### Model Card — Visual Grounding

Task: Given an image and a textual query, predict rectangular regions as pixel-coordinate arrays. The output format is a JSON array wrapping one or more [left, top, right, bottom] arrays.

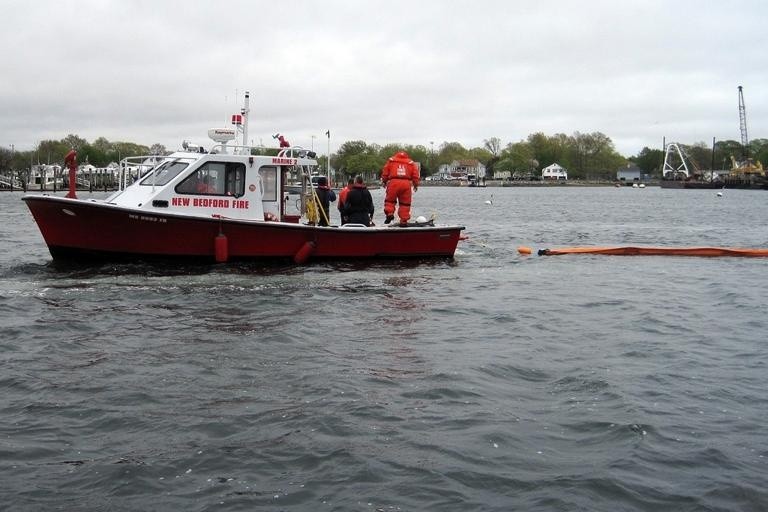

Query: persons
[[199, 175, 216, 195], [315, 150, 420, 227]]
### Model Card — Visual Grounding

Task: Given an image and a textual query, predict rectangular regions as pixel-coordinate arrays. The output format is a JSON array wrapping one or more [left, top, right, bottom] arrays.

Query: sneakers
[[384, 215, 394, 224]]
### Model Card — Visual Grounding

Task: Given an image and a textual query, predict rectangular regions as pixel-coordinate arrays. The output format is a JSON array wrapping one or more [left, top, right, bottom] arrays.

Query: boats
[[278, 172, 341, 194], [19, 88, 471, 264]]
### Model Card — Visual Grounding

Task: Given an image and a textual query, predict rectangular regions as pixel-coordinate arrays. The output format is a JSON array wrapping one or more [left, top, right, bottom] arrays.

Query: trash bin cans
[[467, 174, 476, 181]]
